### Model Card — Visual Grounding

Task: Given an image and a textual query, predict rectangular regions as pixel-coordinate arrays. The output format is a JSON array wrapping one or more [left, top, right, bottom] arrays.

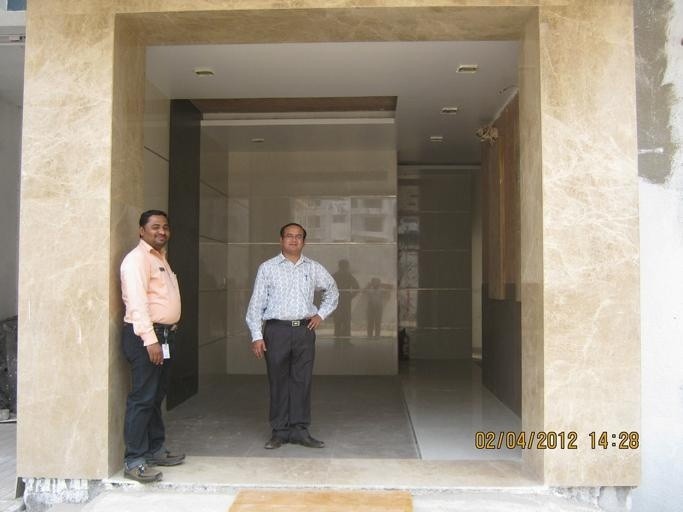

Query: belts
[[291, 321, 302, 328]]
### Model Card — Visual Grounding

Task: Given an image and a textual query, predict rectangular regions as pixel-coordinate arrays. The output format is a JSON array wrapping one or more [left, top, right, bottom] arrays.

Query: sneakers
[[289, 434, 324, 448], [146, 451, 184, 466], [264, 437, 289, 449], [125, 464, 163, 482]]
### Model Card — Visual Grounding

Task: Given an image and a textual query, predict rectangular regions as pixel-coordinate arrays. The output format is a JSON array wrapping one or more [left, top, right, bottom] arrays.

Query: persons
[[119, 209, 185, 483], [245, 222, 340, 449]]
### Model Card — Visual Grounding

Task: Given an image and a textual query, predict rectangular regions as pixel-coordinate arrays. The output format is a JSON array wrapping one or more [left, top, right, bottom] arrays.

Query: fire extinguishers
[[399, 328, 409, 360]]
